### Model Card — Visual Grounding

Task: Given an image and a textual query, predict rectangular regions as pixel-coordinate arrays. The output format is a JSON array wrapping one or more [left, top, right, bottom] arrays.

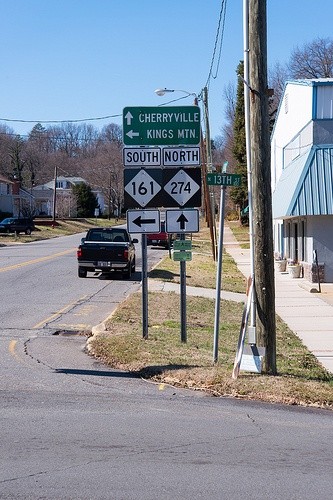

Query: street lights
[[155, 86, 216, 226]]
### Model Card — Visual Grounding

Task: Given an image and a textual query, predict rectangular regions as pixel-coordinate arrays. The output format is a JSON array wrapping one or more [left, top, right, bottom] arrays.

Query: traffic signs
[[122, 105, 203, 147]]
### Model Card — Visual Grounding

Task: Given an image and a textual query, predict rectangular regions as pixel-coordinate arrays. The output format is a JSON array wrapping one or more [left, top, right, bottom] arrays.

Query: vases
[[287, 265, 301, 278], [275, 260, 286, 272]]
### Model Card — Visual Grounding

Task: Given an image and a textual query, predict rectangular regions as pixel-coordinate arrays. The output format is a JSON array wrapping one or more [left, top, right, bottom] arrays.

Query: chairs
[[93, 233, 101, 240], [113, 236, 124, 242]]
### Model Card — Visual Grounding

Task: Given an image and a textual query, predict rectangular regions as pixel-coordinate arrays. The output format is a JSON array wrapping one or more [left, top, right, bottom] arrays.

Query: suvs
[[0, 218, 35, 236], [147, 220, 177, 248]]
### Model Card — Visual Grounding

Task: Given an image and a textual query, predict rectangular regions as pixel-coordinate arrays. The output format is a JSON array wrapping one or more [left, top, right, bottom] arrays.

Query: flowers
[[286, 259, 302, 267], [273, 250, 286, 262]]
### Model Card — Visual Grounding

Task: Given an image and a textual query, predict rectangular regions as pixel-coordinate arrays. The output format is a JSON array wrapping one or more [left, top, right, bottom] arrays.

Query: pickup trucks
[[76, 227, 139, 281]]
